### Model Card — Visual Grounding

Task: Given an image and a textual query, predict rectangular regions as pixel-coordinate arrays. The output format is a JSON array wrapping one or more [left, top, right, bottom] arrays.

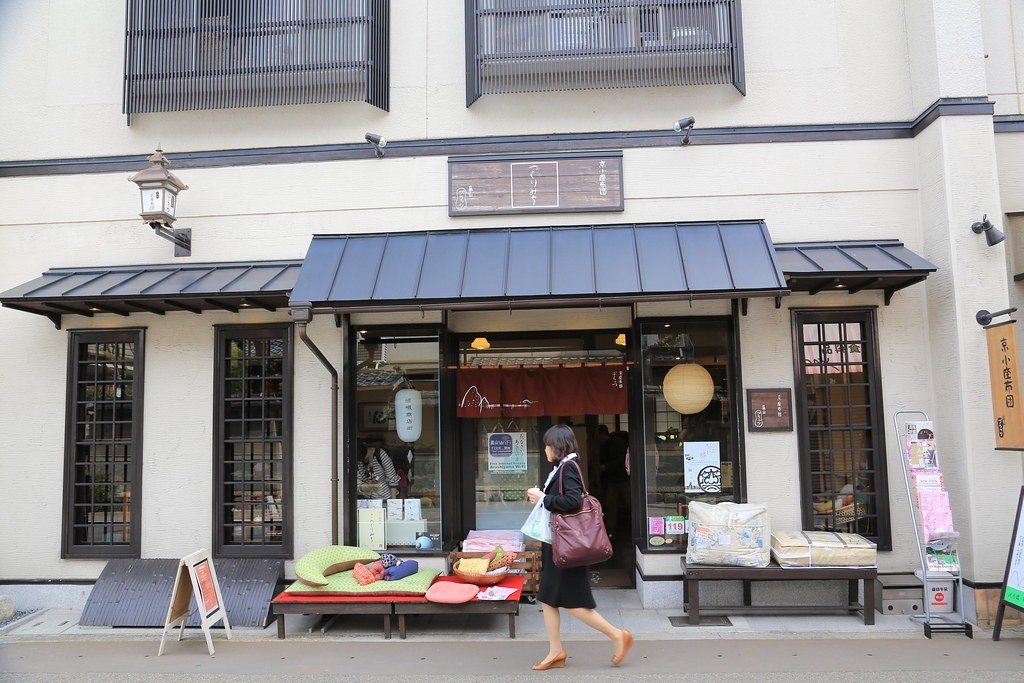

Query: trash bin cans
[[915, 569, 954, 614]]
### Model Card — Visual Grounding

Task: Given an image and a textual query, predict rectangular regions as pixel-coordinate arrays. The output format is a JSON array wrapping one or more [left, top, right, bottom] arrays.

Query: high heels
[[612, 628, 634, 665], [532, 648, 567, 671]]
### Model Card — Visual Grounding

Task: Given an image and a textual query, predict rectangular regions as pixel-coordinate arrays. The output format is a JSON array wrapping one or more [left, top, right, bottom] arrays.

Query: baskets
[[453, 556, 509, 585]]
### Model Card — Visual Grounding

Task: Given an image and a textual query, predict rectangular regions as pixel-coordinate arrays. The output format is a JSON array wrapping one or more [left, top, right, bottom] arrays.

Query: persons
[[527, 424, 633, 671], [357, 442, 401, 503], [598, 425, 627, 530]]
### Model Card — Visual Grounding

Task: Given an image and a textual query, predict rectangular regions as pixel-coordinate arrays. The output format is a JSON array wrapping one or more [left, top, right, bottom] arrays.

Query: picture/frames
[[356, 400, 397, 433]]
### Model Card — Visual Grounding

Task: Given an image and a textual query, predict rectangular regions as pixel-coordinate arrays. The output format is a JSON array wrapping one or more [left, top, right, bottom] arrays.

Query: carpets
[[588, 568, 633, 586]]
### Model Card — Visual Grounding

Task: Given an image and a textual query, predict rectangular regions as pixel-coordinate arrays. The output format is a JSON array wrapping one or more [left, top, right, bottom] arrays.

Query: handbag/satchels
[[521, 496, 554, 545], [552, 459, 614, 568]]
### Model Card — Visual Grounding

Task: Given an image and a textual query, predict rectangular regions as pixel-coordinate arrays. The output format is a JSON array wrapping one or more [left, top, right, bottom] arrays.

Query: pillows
[[294, 545, 380, 585]]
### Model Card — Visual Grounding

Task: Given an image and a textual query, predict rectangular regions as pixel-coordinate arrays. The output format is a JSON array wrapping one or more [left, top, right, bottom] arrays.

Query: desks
[[273, 600, 520, 640], [680, 556, 877, 626]]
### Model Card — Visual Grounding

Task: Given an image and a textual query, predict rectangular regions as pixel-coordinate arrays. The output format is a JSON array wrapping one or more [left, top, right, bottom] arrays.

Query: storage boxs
[[875, 576, 924, 615]]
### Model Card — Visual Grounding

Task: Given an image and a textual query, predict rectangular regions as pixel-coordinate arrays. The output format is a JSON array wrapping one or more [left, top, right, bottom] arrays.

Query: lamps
[[615, 334, 626, 346], [663, 347, 714, 415], [971, 214, 1007, 249], [365, 132, 386, 158], [470, 337, 490, 351], [127, 142, 192, 258], [671, 117, 696, 145]]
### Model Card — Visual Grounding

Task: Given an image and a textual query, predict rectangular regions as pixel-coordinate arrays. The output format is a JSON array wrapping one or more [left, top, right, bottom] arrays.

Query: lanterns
[[663, 364, 714, 414], [395, 386, 422, 446]]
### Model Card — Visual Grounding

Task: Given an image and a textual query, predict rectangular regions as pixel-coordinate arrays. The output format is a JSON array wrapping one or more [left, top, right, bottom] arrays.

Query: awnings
[[289, 219, 791, 328], [0, 258, 304, 330], [774, 238, 941, 306]]
